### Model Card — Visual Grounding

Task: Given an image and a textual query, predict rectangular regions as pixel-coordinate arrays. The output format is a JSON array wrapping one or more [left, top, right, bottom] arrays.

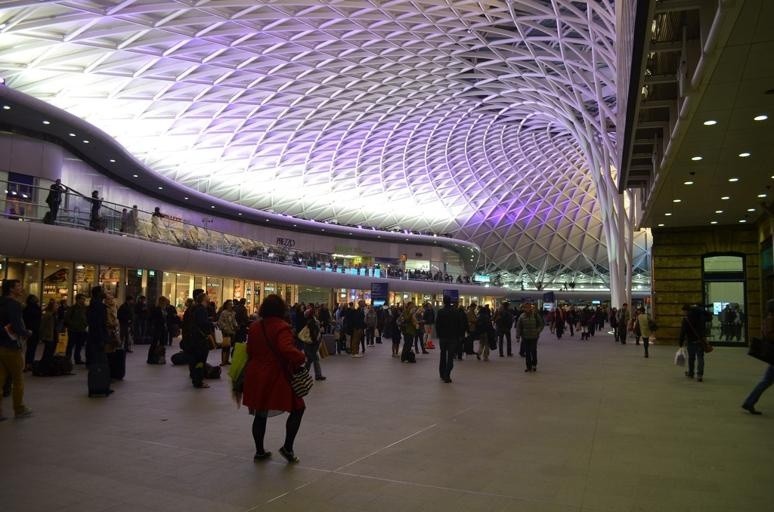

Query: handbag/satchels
[[704, 340, 713, 353], [409, 351, 415, 362], [297, 326, 313, 345], [289, 366, 314, 397]]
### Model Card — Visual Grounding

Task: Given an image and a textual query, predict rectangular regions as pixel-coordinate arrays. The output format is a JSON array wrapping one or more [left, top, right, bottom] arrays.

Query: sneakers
[[254, 451, 271, 460], [278, 446, 299, 463]]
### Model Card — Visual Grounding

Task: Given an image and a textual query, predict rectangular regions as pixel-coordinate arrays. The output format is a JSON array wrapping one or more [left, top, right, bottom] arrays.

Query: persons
[[128, 205, 137, 234], [239, 241, 471, 285], [739, 300, 773, 414], [149, 207, 168, 243], [719, 304, 744, 341], [120, 207, 129, 234], [288, 299, 436, 381], [242, 294, 305, 465], [537, 282, 541, 290], [435, 294, 545, 383], [562, 281, 568, 291], [678, 304, 713, 382], [22, 285, 250, 397], [568, 282, 572, 289], [547, 301, 657, 358], [572, 281, 575, 289], [90, 190, 103, 231], [44, 179, 67, 225], [519, 278, 524, 290], [2, 278, 34, 421]]
